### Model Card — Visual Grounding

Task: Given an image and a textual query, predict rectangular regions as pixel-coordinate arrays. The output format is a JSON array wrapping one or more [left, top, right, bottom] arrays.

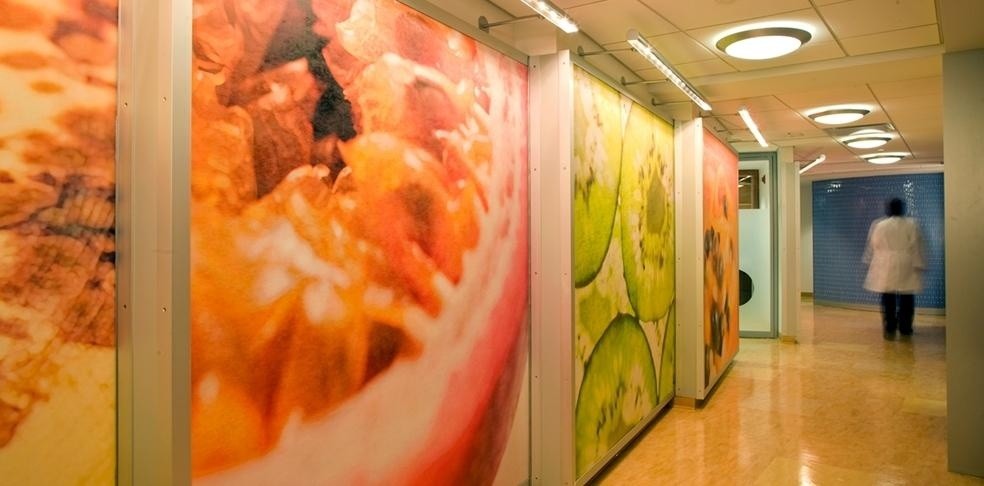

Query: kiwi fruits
[[572, 65, 676, 479]]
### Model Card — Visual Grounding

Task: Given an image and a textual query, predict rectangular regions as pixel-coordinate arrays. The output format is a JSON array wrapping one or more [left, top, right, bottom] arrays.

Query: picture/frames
[[737, 169, 758, 210]]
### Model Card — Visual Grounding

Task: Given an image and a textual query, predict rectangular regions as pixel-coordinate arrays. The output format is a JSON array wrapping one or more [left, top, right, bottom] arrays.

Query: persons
[[863, 197, 928, 341]]
